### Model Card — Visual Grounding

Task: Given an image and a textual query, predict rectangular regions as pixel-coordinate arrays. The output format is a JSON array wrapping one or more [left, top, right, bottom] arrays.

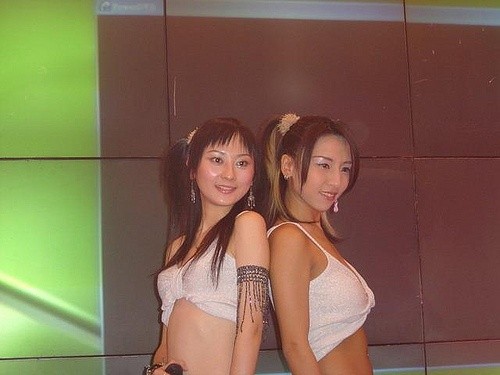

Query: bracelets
[[146, 362, 165, 375]]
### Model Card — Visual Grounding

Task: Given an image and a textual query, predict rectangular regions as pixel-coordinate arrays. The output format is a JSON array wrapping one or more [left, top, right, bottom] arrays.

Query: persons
[[143, 116, 270, 375], [257, 110, 377, 375]]
